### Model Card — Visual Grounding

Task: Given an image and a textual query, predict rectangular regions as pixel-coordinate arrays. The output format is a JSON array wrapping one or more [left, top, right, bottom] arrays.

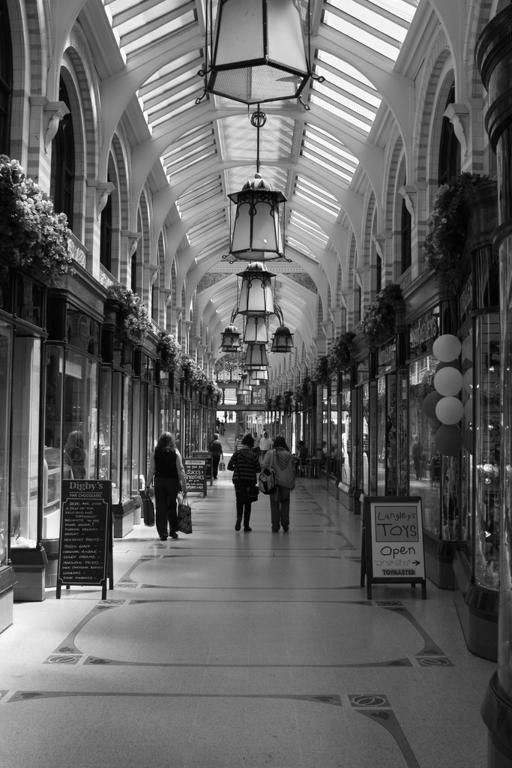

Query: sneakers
[[235, 518, 241, 531], [169, 529, 177, 537], [273, 525, 289, 533], [244, 527, 251, 532], [160, 532, 169, 540]]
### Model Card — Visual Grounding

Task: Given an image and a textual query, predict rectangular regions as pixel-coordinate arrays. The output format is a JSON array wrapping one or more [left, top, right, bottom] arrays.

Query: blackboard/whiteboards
[[366, 497, 427, 582], [183, 451, 213, 492], [57, 479, 111, 585]]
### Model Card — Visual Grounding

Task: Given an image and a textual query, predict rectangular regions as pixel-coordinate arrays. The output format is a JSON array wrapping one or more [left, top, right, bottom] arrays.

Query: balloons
[[432, 333, 462, 365], [421, 362, 463, 458]]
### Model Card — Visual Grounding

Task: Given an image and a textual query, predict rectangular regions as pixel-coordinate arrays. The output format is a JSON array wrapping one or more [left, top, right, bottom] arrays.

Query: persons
[[220, 419, 225, 436], [294, 440, 308, 476], [233, 428, 273, 460], [215, 417, 220, 433], [227, 433, 261, 533], [207, 433, 224, 480], [142, 430, 188, 542], [63, 430, 86, 481], [411, 436, 427, 481], [261, 435, 297, 534], [321, 440, 330, 470]]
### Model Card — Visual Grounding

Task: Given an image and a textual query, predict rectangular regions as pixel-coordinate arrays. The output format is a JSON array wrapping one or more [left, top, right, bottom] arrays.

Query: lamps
[[226, 105, 288, 263], [191, 0, 329, 105], [219, 265, 295, 392]]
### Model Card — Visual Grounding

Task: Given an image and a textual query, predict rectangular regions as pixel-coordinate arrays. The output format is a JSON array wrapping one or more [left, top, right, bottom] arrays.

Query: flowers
[[263, 295, 399, 403], [419, 169, 499, 279], [103, 281, 224, 405], [2, 151, 77, 285]]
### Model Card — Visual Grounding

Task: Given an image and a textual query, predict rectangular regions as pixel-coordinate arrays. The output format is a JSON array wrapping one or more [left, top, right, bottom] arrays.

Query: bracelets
[[145, 486, 150, 488]]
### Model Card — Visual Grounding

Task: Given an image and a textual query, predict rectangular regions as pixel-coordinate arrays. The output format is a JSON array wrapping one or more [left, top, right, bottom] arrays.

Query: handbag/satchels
[[175, 504, 192, 535], [236, 479, 257, 498], [143, 500, 155, 526], [259, 468, 277, 495]]
